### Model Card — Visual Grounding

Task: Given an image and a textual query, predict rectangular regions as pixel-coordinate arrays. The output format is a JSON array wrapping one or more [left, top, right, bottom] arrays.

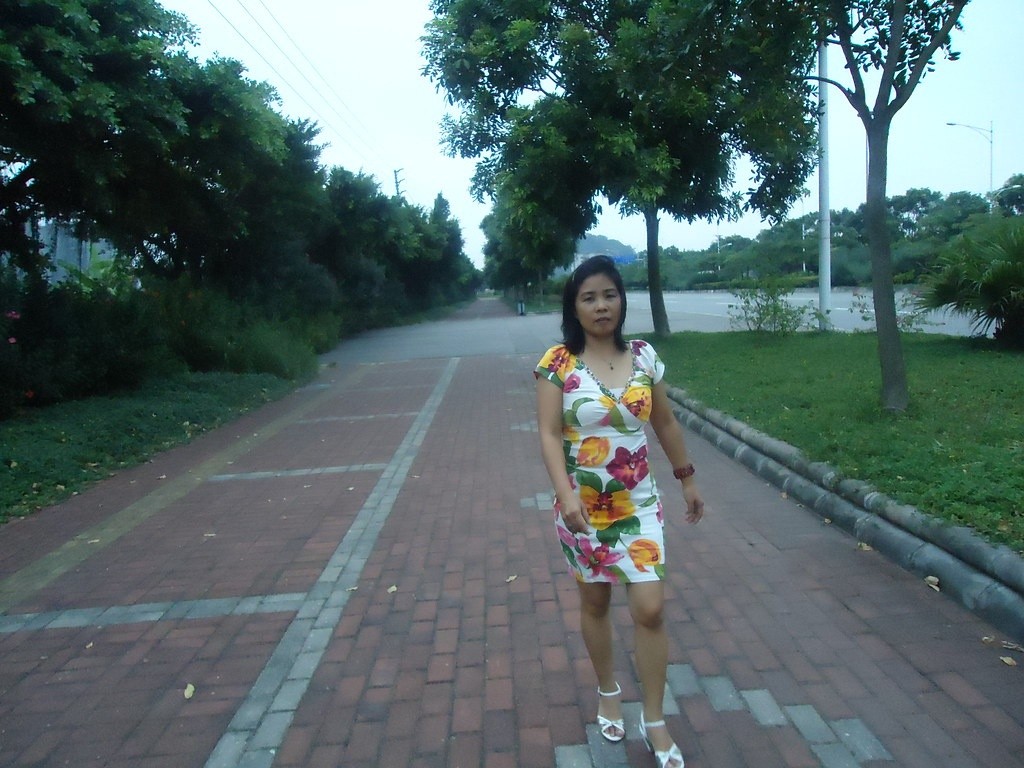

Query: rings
[[567, 524, 572, 527]]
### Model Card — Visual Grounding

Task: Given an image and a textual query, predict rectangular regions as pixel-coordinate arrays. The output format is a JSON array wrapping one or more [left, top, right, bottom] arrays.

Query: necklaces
[[588, 346, 617, 370]]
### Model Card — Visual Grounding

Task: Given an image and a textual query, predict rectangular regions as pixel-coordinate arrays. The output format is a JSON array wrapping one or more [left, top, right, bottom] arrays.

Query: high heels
[[597, 682, 626, 742], [638, 711, 684, 768]]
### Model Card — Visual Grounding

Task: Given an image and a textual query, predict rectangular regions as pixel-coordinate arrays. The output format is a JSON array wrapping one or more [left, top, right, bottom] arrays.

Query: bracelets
[[673, 462, 696, 479]]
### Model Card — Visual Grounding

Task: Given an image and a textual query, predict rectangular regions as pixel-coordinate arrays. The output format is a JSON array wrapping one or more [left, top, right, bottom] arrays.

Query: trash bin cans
[[518, 300, 525, 316]]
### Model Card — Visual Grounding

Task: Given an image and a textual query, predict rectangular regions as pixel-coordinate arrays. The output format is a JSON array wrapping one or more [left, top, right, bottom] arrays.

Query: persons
[[533, 255, 706, 768]]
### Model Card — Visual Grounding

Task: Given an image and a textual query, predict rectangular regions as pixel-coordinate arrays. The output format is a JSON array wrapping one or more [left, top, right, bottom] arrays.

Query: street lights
[[713, 235, 733, 270], [946, 119, 994, 221]]
